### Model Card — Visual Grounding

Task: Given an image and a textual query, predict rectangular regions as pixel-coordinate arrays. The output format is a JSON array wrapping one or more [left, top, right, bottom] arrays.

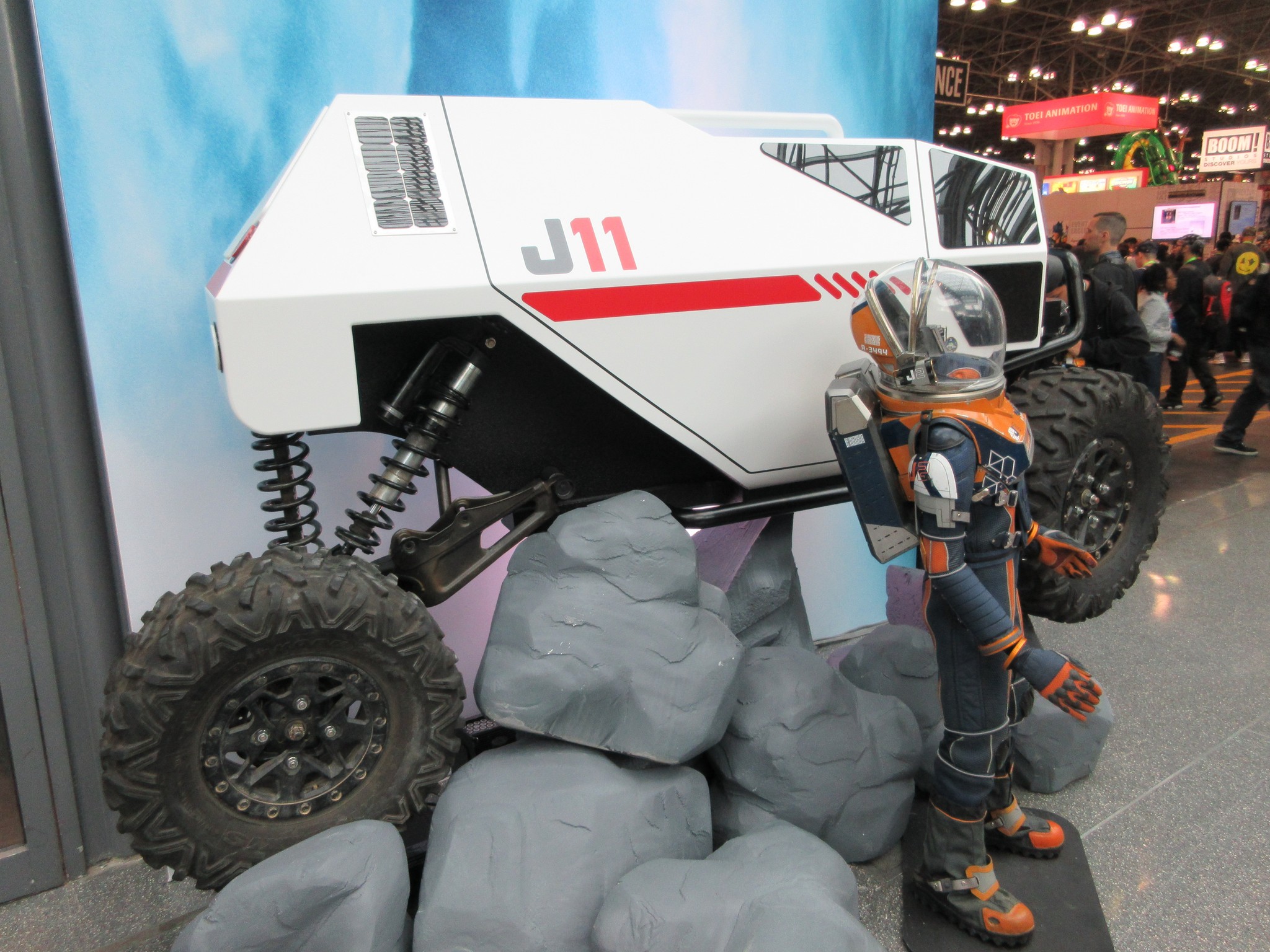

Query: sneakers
[[1212, 431, 1258, 456]]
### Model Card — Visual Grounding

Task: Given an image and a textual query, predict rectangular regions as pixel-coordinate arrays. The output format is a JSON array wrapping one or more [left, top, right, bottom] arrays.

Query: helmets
[[849, 257, 1008, 394]]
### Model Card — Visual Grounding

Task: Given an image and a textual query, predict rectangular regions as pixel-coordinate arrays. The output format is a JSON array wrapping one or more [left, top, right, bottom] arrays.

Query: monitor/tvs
[[1228, 201, 1257, 236], [1149, 202, 1216, 239]]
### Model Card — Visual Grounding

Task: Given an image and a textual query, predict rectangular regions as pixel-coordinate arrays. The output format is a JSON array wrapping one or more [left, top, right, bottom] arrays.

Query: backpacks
[[1180, 261, 1233, 333]]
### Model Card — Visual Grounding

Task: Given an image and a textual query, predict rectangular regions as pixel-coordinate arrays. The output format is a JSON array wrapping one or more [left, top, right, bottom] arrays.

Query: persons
[[824, 257, 1102, 947], [1044, 211, 1270, 456]]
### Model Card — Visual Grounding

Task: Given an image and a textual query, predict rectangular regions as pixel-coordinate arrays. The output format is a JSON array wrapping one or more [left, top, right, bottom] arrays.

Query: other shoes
[[1157, 395, 1184, 409], [1198, 389, 1224, 407], [1208, 352, 1226, 364], [1237, 352, 1250, 363]]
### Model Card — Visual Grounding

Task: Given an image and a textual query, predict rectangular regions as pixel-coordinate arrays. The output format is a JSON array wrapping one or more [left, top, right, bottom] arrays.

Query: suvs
[[92, 86, 1173, 897]]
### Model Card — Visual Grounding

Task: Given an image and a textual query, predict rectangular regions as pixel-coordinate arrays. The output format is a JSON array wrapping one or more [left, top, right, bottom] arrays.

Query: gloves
[[1025, 521, 1098, 579], [1003, 637, 1103, 722]]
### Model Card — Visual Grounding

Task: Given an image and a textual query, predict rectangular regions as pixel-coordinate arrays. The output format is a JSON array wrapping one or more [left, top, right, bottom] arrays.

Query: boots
[[984, 762, 1064, 860], [912, 796, 1035, 947]]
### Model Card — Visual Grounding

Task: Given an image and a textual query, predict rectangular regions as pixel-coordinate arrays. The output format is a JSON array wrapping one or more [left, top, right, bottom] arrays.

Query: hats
[[1242, 227, 1257, 236], [1176, 234, 1206, 247], [1134, 239, 1158, 254]]
